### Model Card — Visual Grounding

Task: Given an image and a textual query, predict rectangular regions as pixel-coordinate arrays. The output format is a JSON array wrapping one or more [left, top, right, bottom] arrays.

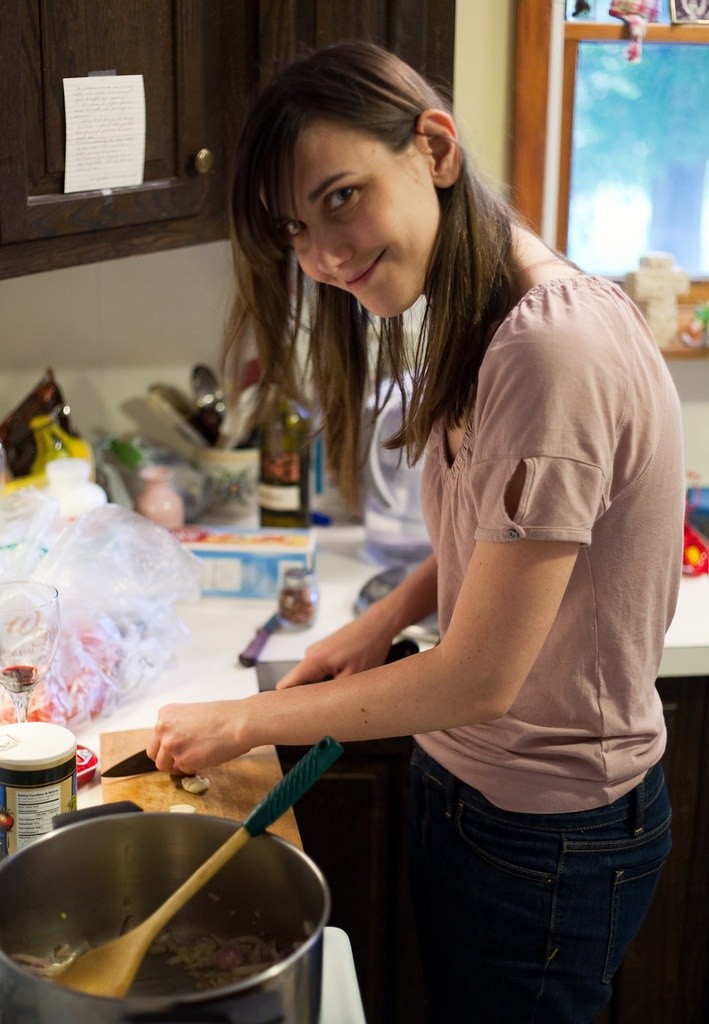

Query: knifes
[[239, 614, 279, 667], [99, 747, 158, 777]]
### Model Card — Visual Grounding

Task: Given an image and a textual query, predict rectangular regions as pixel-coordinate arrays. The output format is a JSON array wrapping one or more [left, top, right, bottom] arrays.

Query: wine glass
[[0, 582, 62, 722]]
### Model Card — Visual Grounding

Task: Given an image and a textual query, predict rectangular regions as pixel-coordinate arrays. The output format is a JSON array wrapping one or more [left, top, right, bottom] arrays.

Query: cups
[[195, 450, 262, 517]]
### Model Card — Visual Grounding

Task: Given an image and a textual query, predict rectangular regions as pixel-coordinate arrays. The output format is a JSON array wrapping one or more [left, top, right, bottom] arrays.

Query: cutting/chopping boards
[[98, 727, 303, 857]]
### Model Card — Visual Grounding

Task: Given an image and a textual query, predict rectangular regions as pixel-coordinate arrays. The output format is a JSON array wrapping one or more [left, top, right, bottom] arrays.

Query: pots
[[0, 800, 332, 1023]]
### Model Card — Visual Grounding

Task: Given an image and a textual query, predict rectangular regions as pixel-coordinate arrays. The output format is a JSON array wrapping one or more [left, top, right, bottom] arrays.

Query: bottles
[[258, 404, 308, 531], [280, 567, 317, 632]]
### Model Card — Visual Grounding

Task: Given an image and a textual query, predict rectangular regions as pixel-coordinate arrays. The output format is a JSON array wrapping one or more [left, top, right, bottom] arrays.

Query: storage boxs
[[169, 525, 319, 599]]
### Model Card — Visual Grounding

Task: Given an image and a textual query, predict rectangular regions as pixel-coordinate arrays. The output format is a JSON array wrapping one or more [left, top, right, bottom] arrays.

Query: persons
[[143, 39, 687, 1024]]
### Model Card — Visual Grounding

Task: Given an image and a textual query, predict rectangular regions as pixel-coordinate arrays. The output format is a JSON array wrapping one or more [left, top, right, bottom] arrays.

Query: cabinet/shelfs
[[276, 672, 709, 1024], [0, 1, 456, 281]]
